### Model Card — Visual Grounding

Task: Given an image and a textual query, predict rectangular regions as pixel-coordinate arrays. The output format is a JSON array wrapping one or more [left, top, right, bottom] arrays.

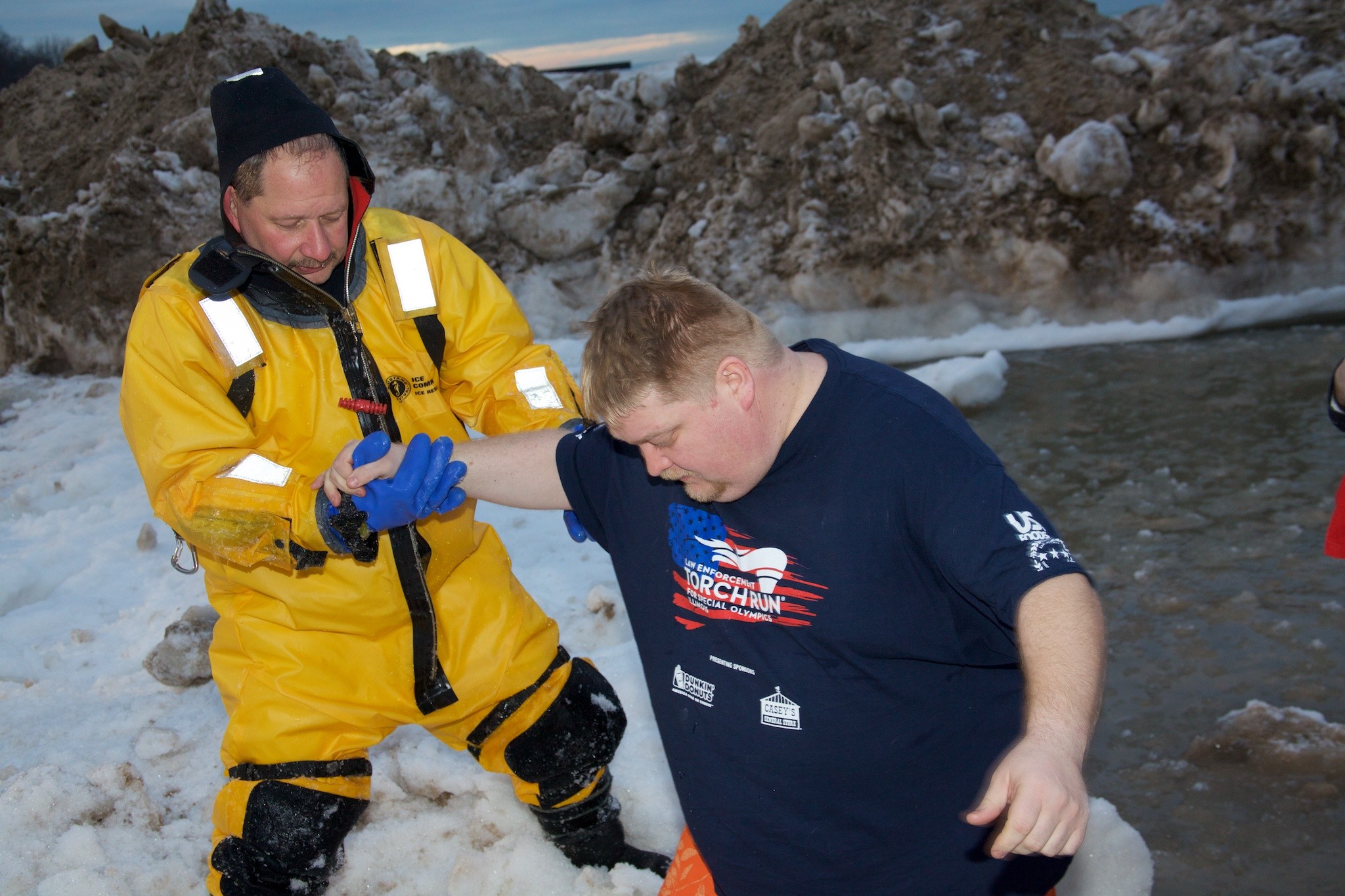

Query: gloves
[[317, 431, 468, 554]]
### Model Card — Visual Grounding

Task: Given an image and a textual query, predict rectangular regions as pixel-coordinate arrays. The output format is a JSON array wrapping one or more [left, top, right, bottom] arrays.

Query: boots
[[531, 772, 672, 880]]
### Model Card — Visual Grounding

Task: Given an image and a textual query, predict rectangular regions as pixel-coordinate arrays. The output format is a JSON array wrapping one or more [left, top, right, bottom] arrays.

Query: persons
[[116, 57, 607, 895], [305, 263, 1113, 895]]
